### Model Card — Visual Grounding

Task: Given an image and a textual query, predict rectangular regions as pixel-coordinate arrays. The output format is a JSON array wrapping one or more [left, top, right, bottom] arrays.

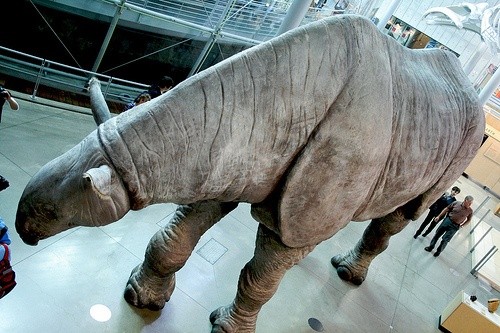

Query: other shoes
[[413, 233, 418, 239], [422, 233, 427, 237], [433, 252, 440, 257], [425, 247, 433, 252]]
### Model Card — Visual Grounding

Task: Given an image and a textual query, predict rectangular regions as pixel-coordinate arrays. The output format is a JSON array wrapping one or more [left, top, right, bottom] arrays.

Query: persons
[[424, 196, 474, 257], [123, 76, 174, 109], [414, 186, 461, 240], [0, 71, 18, 298]]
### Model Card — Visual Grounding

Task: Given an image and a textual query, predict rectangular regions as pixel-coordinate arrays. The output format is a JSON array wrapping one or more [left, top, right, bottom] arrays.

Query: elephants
[[15, 15, 486, 333]]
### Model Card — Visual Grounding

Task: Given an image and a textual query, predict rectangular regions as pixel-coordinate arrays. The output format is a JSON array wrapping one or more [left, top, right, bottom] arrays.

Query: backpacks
[[0, 243, 17, 299]]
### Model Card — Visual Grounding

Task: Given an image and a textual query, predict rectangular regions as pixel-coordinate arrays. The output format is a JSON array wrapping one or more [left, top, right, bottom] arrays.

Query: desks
[[438, 290, 500, 333]]
